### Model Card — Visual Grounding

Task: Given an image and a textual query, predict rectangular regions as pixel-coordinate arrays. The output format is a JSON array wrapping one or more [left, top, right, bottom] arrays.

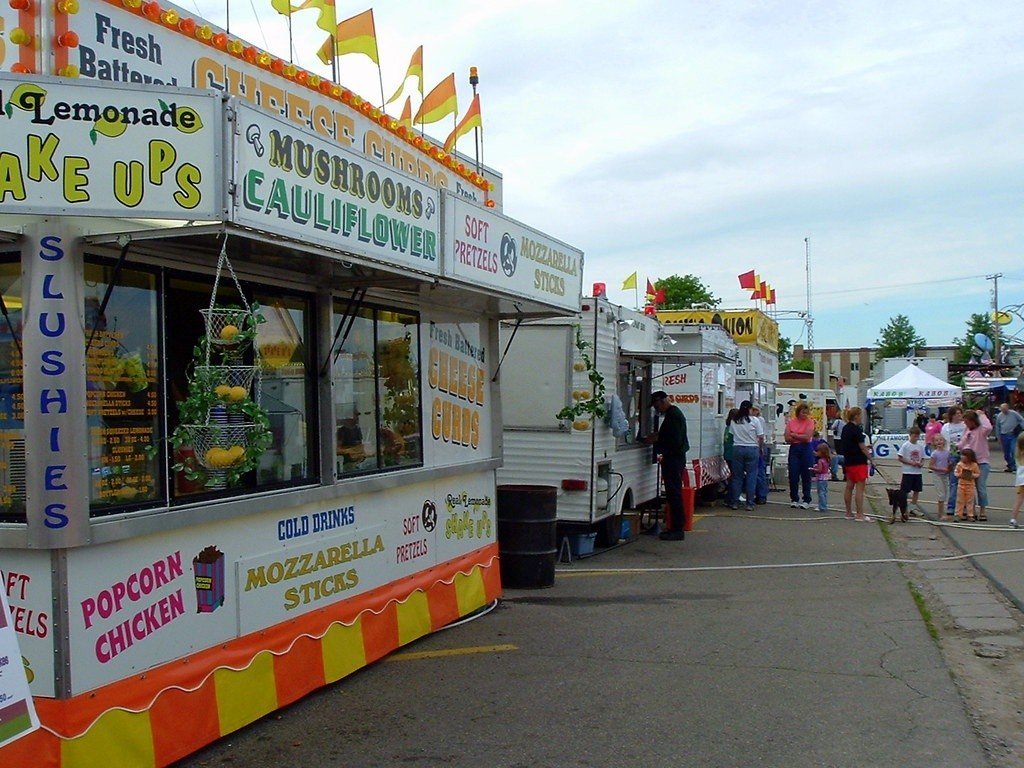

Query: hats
[[647, 390, 668, 410], [753, 401, 761, 411], [740, 401, 753, 409]]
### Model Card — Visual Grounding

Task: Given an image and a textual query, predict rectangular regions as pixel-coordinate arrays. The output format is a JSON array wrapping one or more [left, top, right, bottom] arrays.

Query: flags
[[414, 73, 458, 126], [965, 356, 983, 378], [443, 93, 483, 154], [271, 0, 301, 16], [399, 96, 411, 126], [297, 0, 336, 38], [317, 9, 379, 66], [378, 46, 423, 109]]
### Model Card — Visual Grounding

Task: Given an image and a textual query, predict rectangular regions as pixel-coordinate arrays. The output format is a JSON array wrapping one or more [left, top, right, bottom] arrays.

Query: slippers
[[844, 514, 857, 520], [854, 516, 875, 522]]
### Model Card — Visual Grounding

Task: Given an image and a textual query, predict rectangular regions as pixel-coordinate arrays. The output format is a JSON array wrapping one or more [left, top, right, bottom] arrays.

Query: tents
[[867, 363, 962, 436]]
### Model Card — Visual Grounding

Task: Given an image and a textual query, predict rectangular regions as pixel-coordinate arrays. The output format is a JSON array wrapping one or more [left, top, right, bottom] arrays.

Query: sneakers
[[801, 502, 810, 509], [790, 501, 799, 507]]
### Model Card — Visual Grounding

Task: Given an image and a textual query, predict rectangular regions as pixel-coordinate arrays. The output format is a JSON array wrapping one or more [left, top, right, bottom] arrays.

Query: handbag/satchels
[[834, 430, 838, 435]]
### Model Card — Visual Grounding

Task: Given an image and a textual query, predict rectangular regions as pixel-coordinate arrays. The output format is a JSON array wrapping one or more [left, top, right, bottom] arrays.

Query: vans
[[776, 388, 842, 448]]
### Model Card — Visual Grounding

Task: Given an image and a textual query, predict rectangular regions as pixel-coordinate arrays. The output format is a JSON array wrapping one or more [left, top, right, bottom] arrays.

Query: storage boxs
[[574, 531, 596, 555], [621, 511, 641, 538], [619, 521, 629, 540]]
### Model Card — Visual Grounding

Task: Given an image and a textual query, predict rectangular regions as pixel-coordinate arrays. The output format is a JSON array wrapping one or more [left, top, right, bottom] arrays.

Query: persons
[[397, 418, 419, 460], [723, 400, 768, 511], [784, 404, 874, 522], [896, 403, 1024, 527], [335, 408, 366, 472], [643, 391, 690, 541]]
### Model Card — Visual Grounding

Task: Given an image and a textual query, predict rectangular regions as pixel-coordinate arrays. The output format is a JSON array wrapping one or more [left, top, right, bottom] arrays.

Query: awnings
[[1006, 384, 1018, 390], [962, 384, 1004, 393]]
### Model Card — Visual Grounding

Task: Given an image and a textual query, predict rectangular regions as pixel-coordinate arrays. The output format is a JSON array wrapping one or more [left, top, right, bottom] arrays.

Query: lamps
[[661, 334, 678, 348], [608, 313, 634, 333]]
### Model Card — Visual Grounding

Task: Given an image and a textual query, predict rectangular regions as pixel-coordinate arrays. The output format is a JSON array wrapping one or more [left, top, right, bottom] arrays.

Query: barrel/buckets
[[666, 488, 695, 531], [496, 484, 559, 588]]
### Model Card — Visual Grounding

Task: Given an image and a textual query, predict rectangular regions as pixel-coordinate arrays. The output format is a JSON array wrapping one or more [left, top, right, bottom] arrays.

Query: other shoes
[[1004, 468, 1013, 473], [831, 476, 839, 481], [746, 504, 757, 510], [939, 511, 988, 522], [1009, 518, 1019, 527], [659, 529, 686, 541], [731, 502, 740, 509], [813, 507, 820, 511], [755, 498, 767, 505], [909, 509, 925, 518]]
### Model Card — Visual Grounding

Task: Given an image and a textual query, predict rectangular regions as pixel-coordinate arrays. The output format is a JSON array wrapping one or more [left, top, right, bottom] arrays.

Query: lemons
[[221, 325, 240, 340], [574, 363, 586, 371], [572, 421, 589, 431], [206, 445, 245, 467], [215, 385, 248, 400], [573, 390, 591, 400]]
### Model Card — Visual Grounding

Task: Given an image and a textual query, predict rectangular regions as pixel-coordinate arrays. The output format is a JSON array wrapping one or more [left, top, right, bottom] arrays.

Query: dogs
[[884, 488, 908, 524]]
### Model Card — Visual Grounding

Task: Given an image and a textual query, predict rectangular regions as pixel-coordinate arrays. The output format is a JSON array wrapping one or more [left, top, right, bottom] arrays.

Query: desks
[[871, 432, 931, 458]]
